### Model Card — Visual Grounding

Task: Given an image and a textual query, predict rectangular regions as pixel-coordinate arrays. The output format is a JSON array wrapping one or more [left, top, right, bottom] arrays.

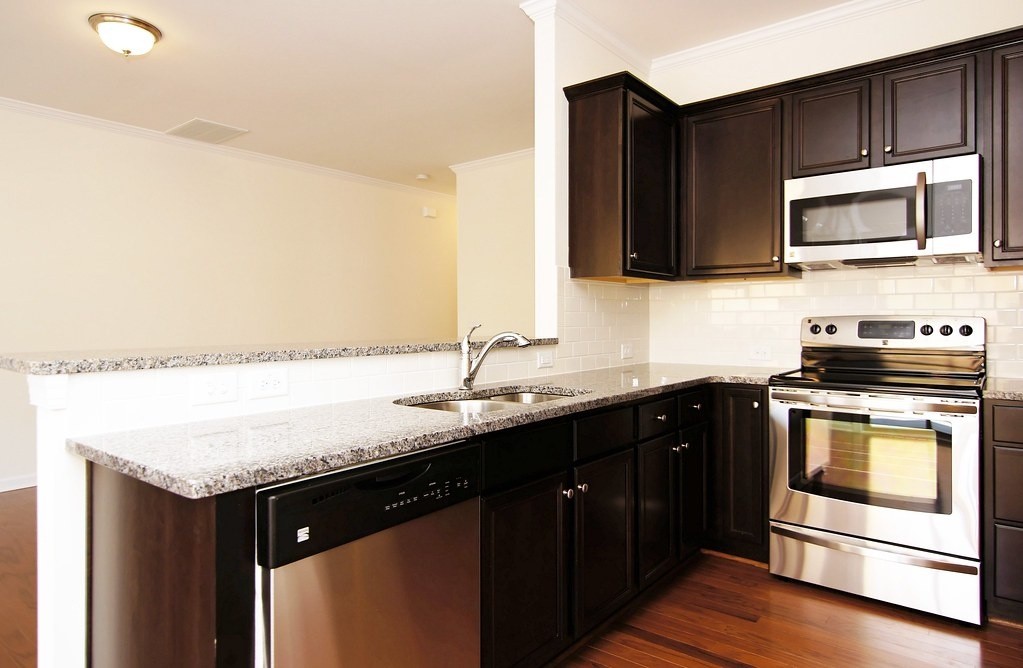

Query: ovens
[[768, 386, 980, 625]]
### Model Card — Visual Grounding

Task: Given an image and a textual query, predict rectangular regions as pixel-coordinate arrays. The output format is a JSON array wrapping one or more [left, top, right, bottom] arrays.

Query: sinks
[[402, 400, 519, 421], [490, 392, 578, 405]]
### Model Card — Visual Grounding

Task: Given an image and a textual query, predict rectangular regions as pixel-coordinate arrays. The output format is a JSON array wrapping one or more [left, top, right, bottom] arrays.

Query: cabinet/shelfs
[[491, 401, 638, 668], [680, 76, 802, 282], [983, 400, 1023, 618], [704, 383, 769, 564], [787, 32, 992, 179], [563, 70, 679, 283], [638, 384, 704, 598], [984, 25, 1023, 267]]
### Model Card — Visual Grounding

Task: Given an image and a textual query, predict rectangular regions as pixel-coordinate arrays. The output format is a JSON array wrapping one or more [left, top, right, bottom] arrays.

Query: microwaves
[[784, 154, 984, 264]]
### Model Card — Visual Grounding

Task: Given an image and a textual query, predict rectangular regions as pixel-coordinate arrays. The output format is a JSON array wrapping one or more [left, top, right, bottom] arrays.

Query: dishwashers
[[249, 437, 481, 668]]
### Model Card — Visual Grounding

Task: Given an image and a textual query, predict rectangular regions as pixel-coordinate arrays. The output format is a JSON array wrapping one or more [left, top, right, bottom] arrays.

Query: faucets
[[458, 324, 531, 390]]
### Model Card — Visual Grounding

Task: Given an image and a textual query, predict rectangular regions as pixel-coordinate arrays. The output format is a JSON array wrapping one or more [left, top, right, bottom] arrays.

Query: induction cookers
[[769, 315, 987, 399]]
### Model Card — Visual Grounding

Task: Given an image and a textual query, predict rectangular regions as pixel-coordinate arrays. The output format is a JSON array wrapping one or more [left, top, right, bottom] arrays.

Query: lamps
[[88, 13, 162, 58]]
